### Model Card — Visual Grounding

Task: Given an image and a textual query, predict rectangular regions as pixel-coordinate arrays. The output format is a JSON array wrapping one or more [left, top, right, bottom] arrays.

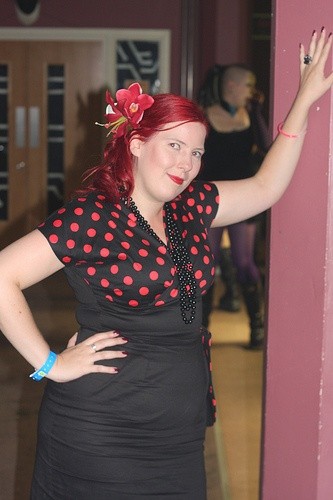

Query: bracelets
[[278, 124, 298, 138], [29, 351, 57, 382]]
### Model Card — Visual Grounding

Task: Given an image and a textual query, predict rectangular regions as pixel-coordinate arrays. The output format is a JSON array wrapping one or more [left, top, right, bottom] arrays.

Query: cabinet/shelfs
[[0, 28, 197, 300]]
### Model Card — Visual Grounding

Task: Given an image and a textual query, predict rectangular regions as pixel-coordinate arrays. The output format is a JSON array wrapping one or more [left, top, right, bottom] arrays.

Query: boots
[[239, 278, 265, 350], [214, 246, 240, 314], [202, 284, 213, 328]]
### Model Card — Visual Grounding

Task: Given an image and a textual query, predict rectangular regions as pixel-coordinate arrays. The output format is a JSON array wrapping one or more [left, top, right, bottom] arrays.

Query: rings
[[304, 56, 312, 65], [92, 344, 96, 350]]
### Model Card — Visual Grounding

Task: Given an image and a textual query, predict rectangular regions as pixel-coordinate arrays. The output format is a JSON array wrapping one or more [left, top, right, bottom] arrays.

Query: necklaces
[[118, 185, 197, 324]]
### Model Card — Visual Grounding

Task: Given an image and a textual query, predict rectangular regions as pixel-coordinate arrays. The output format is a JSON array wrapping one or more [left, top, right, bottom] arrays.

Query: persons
[[198, 63, 265, 349], [0, 25, 333, 500]]
